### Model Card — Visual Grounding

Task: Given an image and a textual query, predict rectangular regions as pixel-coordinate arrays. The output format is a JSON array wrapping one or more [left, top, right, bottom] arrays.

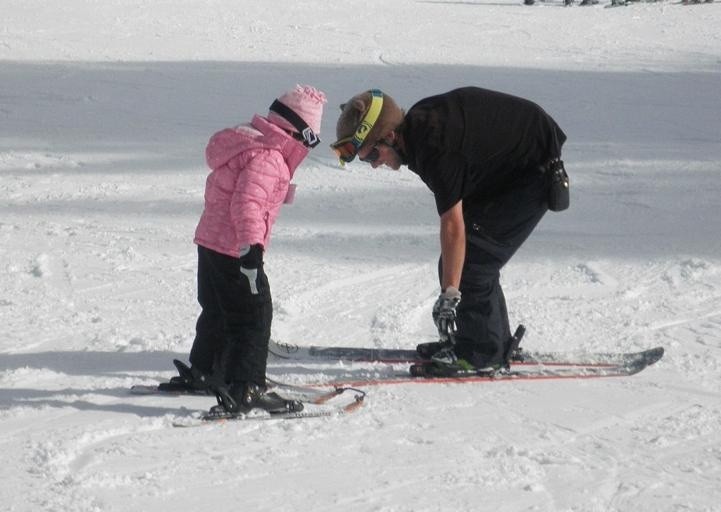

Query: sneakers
[[188, 365, 211, 390], [228, 382, 304, 415], [417, 342, 509, 375]]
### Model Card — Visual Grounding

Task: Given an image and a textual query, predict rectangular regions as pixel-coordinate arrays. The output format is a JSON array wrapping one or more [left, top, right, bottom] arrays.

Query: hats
[[336, 92, 403, 142], [267, 87, 326, 138]]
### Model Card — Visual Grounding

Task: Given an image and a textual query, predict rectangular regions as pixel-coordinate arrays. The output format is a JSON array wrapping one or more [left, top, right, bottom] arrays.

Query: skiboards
[[131, 385, 365, 428], [266, 341, 664, 386]]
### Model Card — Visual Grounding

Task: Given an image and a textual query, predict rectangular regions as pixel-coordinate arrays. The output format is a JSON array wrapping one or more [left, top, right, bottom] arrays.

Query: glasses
[[360, 140, 382, 164], [268, 98, 321, 151], [333, 90, 384, 166]]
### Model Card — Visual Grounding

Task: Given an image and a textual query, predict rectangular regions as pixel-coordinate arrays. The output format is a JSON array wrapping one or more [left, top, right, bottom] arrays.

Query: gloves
[[241, 244, 262, 269], [431, 287, 460, 344]]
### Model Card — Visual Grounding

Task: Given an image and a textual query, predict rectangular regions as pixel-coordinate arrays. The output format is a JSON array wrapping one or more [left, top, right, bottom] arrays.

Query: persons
[[332, 86, 568, 375], [171, 82, 327, 417]]
